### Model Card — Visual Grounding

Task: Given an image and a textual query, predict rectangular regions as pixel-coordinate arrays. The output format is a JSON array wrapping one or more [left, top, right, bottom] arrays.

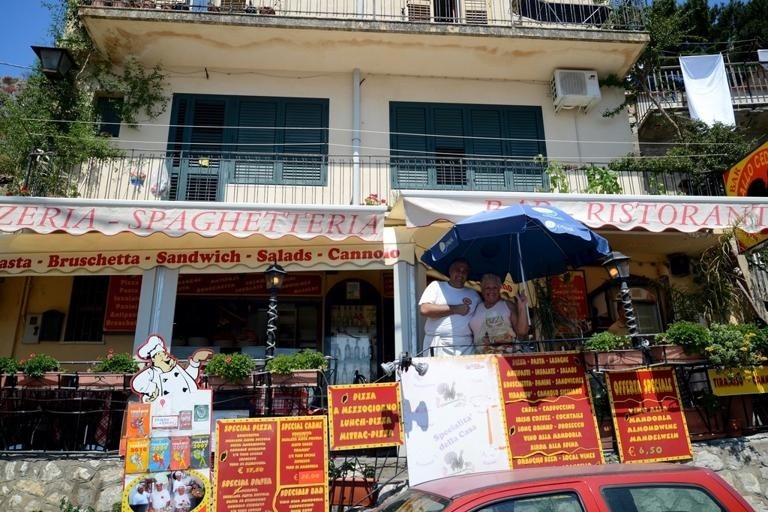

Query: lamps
[[30, 45, 81, 104]]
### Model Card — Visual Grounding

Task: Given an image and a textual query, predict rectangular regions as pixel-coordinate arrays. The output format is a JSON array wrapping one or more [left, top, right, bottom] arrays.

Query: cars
[[344, 462, 756, 512]]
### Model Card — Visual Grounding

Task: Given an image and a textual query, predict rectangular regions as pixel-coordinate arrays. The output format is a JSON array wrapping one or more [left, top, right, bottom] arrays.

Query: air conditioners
[[546, 68, 601, 115]]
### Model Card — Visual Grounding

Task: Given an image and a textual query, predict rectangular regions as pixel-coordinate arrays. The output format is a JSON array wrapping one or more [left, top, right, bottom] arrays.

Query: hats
[[156, 481, 163, 485], [175, 471, 182, 476], [138, 336, 167, 360]]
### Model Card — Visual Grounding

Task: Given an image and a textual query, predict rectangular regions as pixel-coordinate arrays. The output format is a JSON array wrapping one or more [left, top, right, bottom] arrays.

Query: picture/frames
[[101, 275, 142, 335]]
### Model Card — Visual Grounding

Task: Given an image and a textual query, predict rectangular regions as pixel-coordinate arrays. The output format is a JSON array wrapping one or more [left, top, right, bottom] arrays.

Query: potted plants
[[650, 320, 768, 378], [328, 460, 379, 507], [265, 348, 329, 389], [583, 331, 643, 374], [0, 354, 18, 388]]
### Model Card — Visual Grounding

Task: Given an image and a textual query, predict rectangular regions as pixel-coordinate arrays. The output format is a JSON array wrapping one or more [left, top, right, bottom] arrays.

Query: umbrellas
[[421, 204, 609, 342]]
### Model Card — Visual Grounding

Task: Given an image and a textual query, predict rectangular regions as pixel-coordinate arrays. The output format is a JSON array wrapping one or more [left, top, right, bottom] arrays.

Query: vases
[[207, 3, 276, 14], [90, 0, 190, 10], [77, 371, 125, 391], [16, 369, 60, 388], [208, 374, 253, 390]]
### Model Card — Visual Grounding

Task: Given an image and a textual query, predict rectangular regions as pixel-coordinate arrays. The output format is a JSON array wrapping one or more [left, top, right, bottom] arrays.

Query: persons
[[128, 471, 191, 512], [131, 334, 213, 404], [467, 272, 529, 355], [416, 258, 481, 357], [608, 302, 629, 336]]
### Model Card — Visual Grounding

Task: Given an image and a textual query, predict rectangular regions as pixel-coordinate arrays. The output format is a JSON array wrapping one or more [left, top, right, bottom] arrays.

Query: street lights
[[263, 258, 287, 416], [601, 246, 644, 349]]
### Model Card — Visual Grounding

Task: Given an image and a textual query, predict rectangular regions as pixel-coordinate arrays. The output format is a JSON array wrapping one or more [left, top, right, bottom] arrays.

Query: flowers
[[202, 351, 255, 381], [76, 352, 139, 374], [13, 353, 66, 374]]
